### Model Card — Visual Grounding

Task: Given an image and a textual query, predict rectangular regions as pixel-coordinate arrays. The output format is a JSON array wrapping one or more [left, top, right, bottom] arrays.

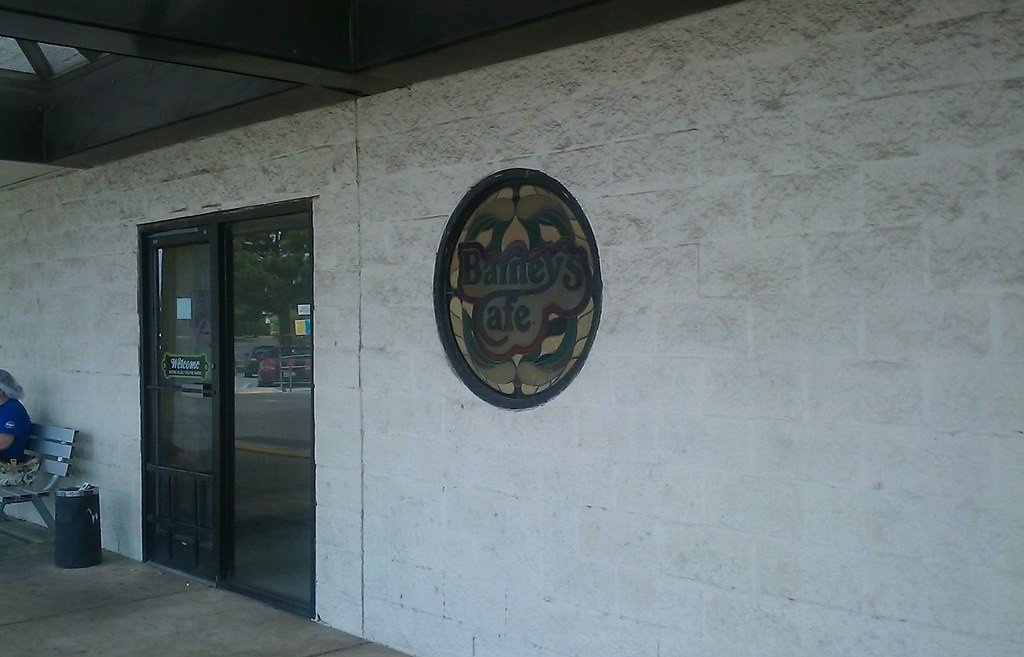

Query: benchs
[[0, 420, 81, 536]]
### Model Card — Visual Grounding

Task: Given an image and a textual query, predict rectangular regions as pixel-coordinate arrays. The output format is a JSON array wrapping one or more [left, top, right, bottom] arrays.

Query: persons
[[0, 369, 32, 487]]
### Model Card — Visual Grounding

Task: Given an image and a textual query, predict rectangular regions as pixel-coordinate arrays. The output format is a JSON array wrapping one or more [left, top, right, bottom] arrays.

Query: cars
[[240, 344, 314, 387]]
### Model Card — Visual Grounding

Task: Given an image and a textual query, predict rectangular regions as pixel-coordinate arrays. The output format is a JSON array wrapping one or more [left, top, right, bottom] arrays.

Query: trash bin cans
[[54, 483, 102, 570]]
